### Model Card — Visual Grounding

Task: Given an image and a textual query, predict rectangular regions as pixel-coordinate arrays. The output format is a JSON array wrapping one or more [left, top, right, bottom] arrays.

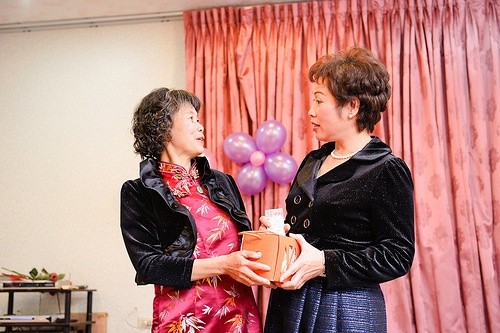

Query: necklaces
[[331, 139, 371, 159], [197, 187, 202, 192]]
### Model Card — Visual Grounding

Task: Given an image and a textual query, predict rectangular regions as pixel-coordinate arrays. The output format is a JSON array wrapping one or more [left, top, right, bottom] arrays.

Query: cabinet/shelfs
[[0, 285, 97, 333]]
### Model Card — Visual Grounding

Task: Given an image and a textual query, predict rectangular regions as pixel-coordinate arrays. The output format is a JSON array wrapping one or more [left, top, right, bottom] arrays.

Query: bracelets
[[320, 251, 326, 277]]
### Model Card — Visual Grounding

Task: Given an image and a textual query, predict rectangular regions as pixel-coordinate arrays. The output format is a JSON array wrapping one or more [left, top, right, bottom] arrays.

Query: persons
[[120, 88, 276, 333], [258, 47, 415, 333]]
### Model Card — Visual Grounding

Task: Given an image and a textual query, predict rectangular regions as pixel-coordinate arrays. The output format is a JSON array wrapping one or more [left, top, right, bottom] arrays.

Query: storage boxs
[[240, 231, 299, 284]]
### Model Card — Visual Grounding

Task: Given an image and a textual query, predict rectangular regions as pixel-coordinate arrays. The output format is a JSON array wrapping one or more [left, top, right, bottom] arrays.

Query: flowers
[[1, 266, 65, 282]]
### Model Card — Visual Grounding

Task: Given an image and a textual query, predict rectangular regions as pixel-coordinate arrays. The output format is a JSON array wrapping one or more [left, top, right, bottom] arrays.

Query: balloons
[[236, 163, 267, 195], [200, 148, 215, 168], [255, 119, 286, 154], [250, 151, 265, 165], [264, 153, 298, 184], [225, 131, 256, 163]]
